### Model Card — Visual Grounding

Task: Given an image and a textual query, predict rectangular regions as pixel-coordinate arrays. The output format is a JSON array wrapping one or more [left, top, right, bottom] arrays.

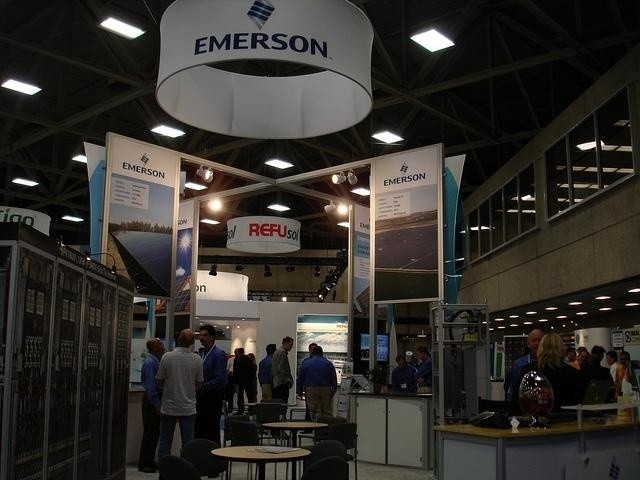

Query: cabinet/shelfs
[[1, 220, 137, 480], [428, 299, 491, 477]]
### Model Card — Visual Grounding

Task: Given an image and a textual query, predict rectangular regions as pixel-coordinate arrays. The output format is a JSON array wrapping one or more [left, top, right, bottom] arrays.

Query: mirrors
[[519, 369, 555, 432]]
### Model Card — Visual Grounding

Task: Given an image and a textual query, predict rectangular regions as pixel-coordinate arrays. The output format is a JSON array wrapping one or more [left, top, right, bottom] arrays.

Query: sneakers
[[139, 462, 158, 473]]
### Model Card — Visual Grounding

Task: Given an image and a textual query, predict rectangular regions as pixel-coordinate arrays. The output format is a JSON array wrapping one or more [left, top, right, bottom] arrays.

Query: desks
[[433, 413, 640, 480]]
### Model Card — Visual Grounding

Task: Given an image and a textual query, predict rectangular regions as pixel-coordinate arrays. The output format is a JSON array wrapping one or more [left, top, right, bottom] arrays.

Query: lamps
[[208, 261, 342, 302], [329, 166, 358, 187], [323, 196, 337, 213], [194, 162, 216, 183]]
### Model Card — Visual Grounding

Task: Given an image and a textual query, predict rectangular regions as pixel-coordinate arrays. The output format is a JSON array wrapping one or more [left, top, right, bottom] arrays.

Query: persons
[[137, 324, 338, 474], [503, 328, 637, 415], [389, 346, 432, 397]]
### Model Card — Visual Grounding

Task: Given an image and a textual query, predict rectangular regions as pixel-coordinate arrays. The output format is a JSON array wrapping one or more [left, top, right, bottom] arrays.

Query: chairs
[[156, 395, 362, 480]]
